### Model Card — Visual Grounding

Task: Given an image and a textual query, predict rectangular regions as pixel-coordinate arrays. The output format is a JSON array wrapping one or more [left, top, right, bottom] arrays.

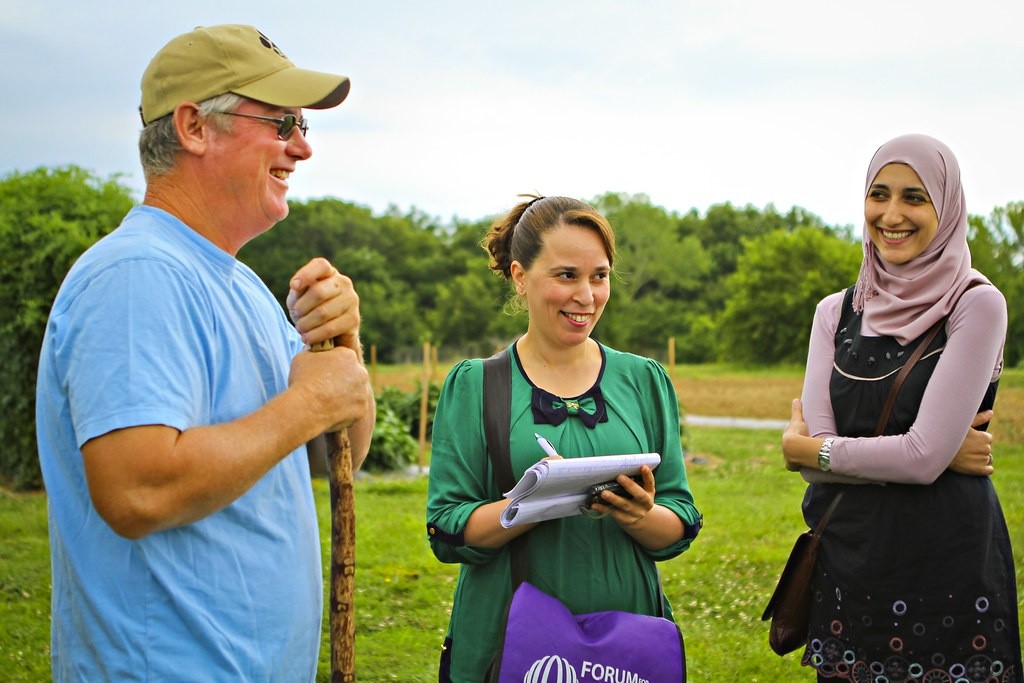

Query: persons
[[780, 134, 1024, 683], [427, 195, 703, 683], [37, 24, 377, 683]]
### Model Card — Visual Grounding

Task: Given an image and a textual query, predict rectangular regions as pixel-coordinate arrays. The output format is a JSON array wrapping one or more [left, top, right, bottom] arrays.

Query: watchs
[[818, 437, 834, 472]]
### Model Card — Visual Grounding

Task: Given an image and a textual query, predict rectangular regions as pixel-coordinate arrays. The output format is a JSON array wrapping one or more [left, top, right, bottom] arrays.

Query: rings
[[988, 454, 993, 465]]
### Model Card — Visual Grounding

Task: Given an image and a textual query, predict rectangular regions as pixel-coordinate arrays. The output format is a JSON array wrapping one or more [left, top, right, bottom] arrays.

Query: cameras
[[584, 476, 641, 510]]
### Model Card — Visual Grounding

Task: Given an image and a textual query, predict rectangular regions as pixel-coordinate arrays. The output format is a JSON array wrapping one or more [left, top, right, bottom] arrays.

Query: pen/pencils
[[533, 431, 558, 458]]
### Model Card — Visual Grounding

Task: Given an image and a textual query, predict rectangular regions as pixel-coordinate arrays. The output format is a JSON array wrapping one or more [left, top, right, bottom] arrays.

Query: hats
[[139, 25, 350, 128]]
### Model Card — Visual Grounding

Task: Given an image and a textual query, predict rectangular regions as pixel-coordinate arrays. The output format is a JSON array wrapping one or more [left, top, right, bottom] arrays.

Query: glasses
[[206, 110, 308, 141]]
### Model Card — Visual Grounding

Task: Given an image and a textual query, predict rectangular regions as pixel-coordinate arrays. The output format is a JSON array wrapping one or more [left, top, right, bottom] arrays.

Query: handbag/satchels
[[762, 530, 821, 657], [483, 582, 686, 683]]
